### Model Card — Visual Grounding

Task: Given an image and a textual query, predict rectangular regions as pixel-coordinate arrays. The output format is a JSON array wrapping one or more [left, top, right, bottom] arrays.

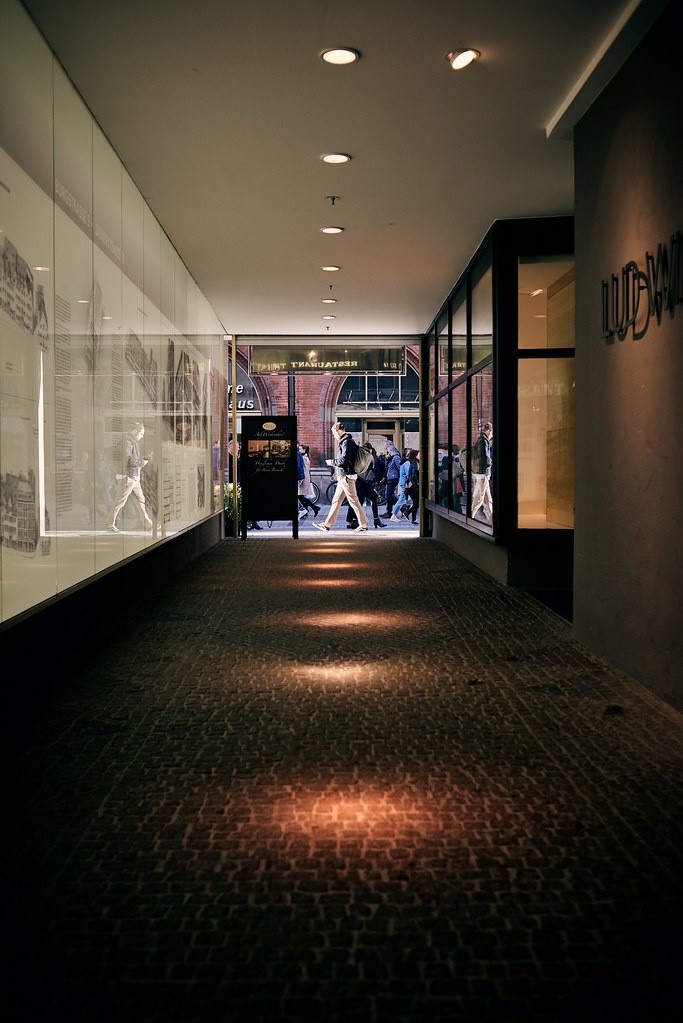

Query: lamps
[[449, 49, 475, 70]]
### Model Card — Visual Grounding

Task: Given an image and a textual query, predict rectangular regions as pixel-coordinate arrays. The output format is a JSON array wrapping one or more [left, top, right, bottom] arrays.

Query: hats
[[362, 443, 372, 449]]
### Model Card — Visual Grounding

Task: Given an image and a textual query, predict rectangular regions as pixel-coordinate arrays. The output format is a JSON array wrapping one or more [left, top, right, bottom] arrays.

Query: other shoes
[[314, 506, 322, 517], [347, 522, 357, 528], [410, 520, 419, 525], [402, 512, 410, 522], [379, 512, 393, 520]]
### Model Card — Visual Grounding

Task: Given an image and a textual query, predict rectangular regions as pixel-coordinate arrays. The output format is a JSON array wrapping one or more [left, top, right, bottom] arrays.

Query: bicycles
[[325, 480, 389, 507], [299, 480, 320, 506]]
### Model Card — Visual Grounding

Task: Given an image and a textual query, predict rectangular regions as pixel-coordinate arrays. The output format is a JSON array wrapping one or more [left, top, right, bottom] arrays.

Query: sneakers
[[356, 522, 368, 531], [391, 514, 401, 523], [311, 522, 329, 532], [299, 509, 308, 519]]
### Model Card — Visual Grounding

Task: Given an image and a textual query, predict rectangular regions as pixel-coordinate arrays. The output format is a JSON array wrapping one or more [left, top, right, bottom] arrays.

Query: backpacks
[[353, 444, 374, 475]]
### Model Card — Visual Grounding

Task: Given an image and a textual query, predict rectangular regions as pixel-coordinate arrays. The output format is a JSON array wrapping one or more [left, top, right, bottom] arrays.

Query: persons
[[346, 439, 420, 530], [211, 438, 321, 531], [439, 422, 493, 520], [105, 422, 162, 532], [312, 422, 370, 532]]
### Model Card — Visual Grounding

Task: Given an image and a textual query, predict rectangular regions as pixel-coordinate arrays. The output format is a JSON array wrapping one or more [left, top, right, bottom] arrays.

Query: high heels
[[374, 518, 387, 528]]
